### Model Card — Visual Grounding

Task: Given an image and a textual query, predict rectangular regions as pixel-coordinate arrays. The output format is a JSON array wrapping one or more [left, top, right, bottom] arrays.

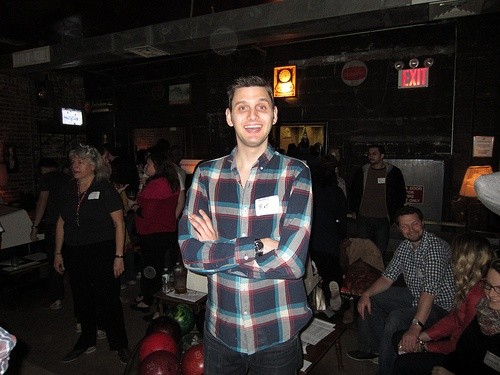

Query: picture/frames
[[2, 141, 19, 173], [272, 65, 298, 99]]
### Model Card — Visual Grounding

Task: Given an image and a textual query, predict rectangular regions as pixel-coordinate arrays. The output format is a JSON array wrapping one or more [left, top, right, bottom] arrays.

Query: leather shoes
[[142, 312, 160, 321], [130, 302, 150, 313]]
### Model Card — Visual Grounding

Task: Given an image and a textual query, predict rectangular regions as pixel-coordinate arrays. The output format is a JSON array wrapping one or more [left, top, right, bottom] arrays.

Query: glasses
[[480, 279, 500, 294]]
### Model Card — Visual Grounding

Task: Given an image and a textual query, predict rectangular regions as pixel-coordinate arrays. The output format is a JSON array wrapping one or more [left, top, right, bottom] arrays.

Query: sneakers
[[48, 302, 62, 310], [329, 280, 341, 311], [97, 330, 107, 340], [60, 339, 96, 364], [74, 323, 82, 333], [116, 346, 131, 365], [346, 350, 379, 362]]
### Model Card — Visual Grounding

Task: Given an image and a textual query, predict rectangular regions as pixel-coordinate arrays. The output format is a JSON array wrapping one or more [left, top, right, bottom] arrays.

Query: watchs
[[254, 239, 264, 257], [413, 319, 424, 328]]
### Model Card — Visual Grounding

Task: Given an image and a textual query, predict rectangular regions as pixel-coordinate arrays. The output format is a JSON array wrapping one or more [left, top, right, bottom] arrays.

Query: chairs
[[334, 238, 386, 330]]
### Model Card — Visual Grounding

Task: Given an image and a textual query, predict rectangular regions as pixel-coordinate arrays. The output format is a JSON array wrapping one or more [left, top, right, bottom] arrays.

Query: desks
[[0, 204, 41, 272]]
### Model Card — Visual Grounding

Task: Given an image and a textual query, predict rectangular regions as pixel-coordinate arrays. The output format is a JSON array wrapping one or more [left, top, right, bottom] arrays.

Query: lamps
[[459, 165, 492, 198]]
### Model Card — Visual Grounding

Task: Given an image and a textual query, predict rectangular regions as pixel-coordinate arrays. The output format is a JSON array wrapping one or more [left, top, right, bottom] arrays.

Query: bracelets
[[115, 255, 123, 258], [420, 339, 427, 352], [55, 253, 62, 255], [31, 226, 40, 229]]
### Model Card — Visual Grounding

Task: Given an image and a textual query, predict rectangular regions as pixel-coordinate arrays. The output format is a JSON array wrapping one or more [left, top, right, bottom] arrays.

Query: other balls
[[139, 303, 205, 375]]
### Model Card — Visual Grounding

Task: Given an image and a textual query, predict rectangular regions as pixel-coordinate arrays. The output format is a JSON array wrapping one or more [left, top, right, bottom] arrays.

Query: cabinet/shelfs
[[154, 272, 362, 375]]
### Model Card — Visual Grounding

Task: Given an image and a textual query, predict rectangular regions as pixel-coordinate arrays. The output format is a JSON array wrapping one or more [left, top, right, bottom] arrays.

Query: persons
[[346, 205, 457, 369], [266, 135, 324, 168], [107, 163, 129, 215], [75, 147, 135, 340], [431, 257, 500, 375], [354, 144, 406, 268], [390, 232, 496, 375], [309, 163, 348, 312], [124, 153, 181, 275], [178, 77, 312, 375], [157, 140, 185, 219], [53, 144, 132, 364], [30, 158, 74, 309]]
